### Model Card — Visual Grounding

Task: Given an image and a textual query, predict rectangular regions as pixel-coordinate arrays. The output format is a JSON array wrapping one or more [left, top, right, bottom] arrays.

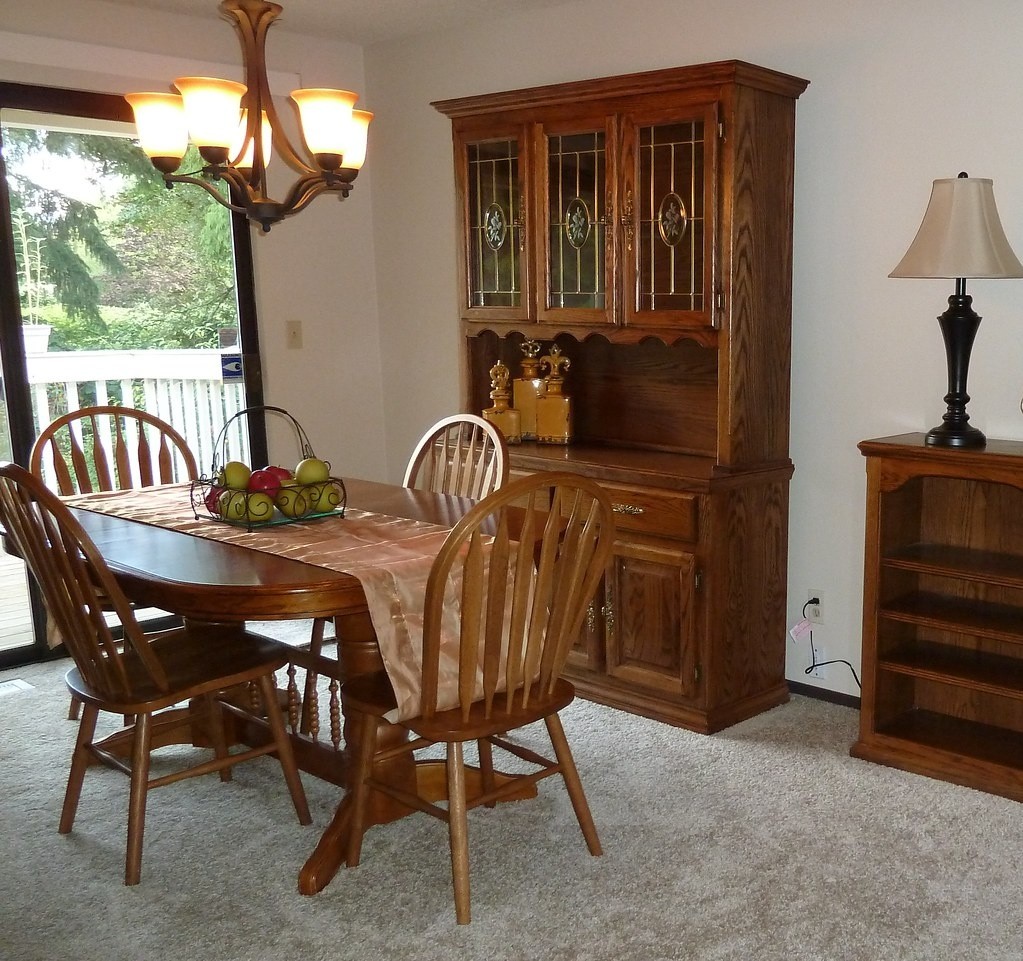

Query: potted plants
[[11, 208, 55, 353]]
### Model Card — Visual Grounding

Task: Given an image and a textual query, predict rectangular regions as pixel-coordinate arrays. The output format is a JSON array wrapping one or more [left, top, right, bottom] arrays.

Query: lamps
[[124, 0, 376, 232], [888, 172, 1023, 447]]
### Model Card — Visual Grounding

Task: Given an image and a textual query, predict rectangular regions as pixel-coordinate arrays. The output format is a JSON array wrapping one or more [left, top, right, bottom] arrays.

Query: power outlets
[[808, 588, 827, 626], [284, 320, 303, 349]]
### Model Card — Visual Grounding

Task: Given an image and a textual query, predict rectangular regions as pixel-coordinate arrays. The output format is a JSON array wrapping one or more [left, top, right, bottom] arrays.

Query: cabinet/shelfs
[[849, 432, 1023, 804], [427, 55, 811, 737]]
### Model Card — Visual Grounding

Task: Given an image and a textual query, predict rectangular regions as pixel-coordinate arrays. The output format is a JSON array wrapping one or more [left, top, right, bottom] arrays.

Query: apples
[[207, 459, 340, 521]]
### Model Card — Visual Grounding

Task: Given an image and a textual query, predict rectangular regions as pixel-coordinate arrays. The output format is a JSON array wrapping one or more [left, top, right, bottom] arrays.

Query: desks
[[0, 471, 579, 895]]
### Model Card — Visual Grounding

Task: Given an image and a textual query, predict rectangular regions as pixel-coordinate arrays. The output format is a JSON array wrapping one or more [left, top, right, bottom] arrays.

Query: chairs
[[32, 407, 245, 719], [0, 460, 313, 886], [399, 414, 509, 499], [345, 471, 604, 925]]
